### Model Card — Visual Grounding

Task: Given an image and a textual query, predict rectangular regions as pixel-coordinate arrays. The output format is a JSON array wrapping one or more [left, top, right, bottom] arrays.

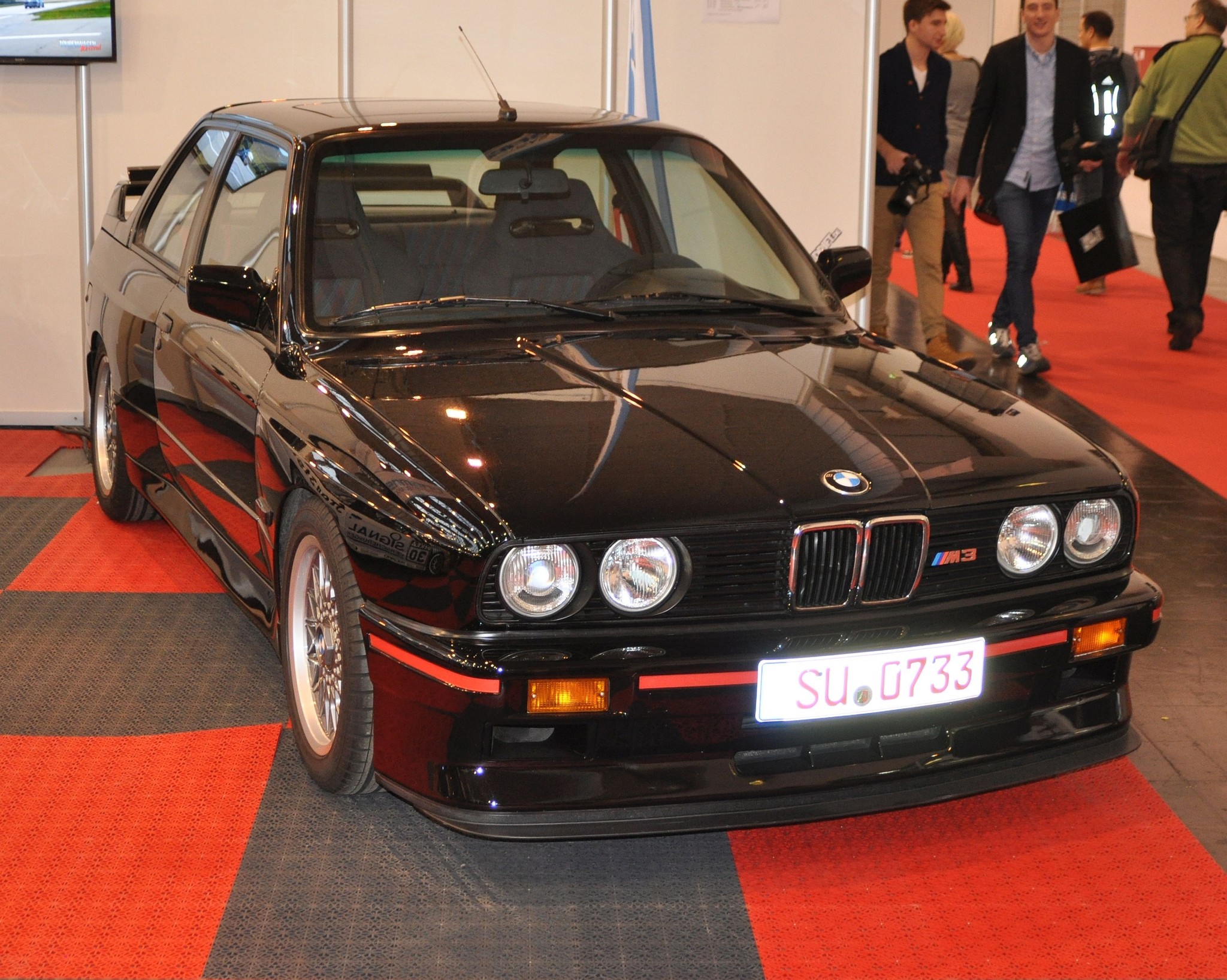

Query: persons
[[1066, 9, 1141, 296], [1113, 0, 1227, 351], [927, 11, 997, 293], [949, 0, 1105, 376], [865, 0, 980, 370]]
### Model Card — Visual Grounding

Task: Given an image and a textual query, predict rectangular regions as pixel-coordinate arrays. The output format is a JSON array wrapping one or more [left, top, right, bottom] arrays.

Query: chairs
[[303, 192, 396, 324], [489, 174, 648, 304]]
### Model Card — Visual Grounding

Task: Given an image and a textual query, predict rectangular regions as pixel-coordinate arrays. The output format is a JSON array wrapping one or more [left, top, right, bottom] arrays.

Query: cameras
[[890, 158, 933, 217]]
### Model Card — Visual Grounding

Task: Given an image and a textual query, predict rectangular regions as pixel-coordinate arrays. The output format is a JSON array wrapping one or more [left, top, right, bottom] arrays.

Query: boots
[[926, 334, 977, 371]]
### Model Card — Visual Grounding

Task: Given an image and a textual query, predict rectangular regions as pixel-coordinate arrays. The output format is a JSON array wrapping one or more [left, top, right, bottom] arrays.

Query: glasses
[[1184, 12, 1200, 23]]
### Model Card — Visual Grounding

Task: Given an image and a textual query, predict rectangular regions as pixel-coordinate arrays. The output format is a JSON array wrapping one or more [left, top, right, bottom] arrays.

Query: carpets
[[2, 720, 303, 978], [732, 760, 1225, 980], [2, 424, 231, 594]]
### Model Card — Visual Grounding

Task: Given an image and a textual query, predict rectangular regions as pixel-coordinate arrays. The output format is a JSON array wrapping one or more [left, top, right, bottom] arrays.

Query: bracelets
[[1116, 141, 1137, 151]]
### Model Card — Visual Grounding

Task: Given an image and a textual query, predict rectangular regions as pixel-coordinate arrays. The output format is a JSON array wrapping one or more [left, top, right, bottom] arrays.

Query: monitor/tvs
[[0, 0, 116, 69]]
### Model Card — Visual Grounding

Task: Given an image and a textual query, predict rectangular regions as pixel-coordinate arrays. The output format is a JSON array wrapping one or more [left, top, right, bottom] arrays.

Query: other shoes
[[949, 282, 973, 293], [1074, 279, 1108, 296], [1167, 311, 1205, 352]]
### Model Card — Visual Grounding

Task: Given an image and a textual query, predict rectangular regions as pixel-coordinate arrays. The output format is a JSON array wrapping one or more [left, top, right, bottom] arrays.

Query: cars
[[85, 21, 1165, 846]]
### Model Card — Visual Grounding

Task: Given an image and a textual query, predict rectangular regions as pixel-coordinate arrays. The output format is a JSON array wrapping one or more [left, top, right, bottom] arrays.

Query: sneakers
[[986, 321, 1016, 360], [1015, 342, 1052, 376]]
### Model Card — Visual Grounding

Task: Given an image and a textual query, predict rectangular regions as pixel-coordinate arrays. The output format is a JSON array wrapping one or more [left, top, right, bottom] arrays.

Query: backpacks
[[1088, 46, 1131, 142]]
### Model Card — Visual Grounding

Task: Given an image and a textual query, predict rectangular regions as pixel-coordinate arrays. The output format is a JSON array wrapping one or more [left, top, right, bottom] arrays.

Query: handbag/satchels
[[1134, 114, 1179, 181], [1057, 193, 1140, 283]]
